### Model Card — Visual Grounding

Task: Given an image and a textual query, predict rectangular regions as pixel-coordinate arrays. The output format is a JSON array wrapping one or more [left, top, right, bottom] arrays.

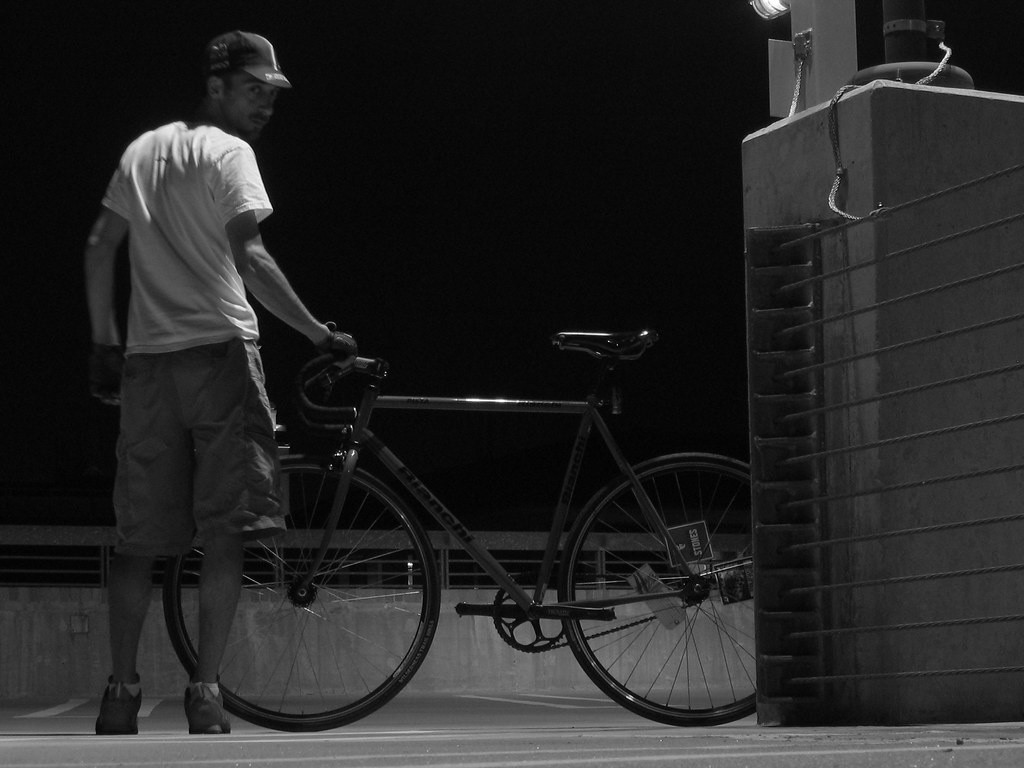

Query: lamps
[[751, 0, 789, 21]]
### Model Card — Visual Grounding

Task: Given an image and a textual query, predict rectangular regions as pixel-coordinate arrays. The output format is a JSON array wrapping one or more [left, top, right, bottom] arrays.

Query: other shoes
[[95, 683, 142, 734], [184, 685, 231, 734]]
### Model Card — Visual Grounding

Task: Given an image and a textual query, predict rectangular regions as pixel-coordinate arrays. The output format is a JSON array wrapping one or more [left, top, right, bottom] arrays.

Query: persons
[[86, 31, 359, 734]]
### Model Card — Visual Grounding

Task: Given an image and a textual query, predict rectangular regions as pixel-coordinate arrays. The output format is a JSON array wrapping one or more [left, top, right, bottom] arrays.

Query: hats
[[208, 31, 291, 88]]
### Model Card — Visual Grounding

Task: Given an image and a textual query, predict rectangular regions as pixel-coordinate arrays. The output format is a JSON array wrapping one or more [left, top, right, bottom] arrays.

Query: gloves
[[317, 332, 357, 373]]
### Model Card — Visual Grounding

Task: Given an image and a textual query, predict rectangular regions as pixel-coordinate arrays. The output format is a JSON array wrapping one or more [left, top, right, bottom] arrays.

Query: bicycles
[[162, 314, 763, 727]]
